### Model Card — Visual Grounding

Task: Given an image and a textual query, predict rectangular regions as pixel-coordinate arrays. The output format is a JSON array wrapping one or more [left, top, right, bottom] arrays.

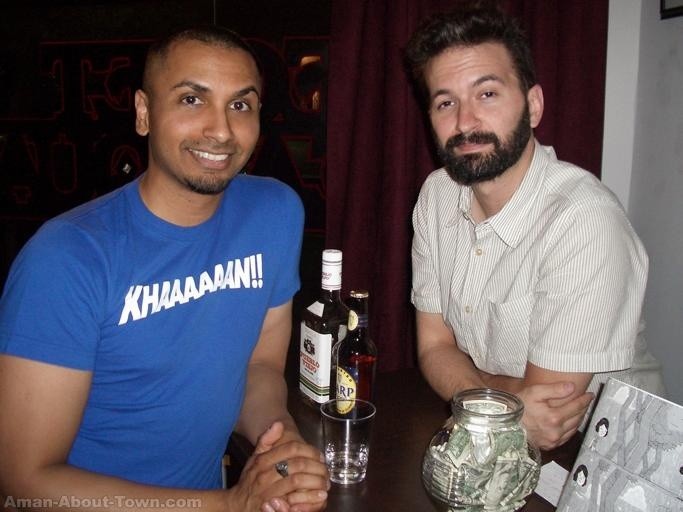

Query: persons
[[402, 8, 667, 451], [0, 25, 332, 512]]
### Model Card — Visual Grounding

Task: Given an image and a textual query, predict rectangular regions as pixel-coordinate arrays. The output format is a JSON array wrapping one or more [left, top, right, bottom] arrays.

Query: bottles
[[335, 289, 377, 422], [419, 389, 542, 512], [298, 248, 356, 416]]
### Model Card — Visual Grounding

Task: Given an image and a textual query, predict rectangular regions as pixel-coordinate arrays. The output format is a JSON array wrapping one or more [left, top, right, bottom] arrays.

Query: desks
[[218, 371, 582, 512]]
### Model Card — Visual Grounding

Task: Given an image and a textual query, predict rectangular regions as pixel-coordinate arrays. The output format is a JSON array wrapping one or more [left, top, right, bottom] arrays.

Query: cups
[[319, 398, 377, 486]]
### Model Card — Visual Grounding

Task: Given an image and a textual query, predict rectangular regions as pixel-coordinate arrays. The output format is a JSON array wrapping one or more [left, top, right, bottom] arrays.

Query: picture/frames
[[657, 0, 683, 20]]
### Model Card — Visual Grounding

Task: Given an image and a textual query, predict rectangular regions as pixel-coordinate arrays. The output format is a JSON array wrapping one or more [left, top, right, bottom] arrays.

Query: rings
[[275, 460, 288, 477]]
